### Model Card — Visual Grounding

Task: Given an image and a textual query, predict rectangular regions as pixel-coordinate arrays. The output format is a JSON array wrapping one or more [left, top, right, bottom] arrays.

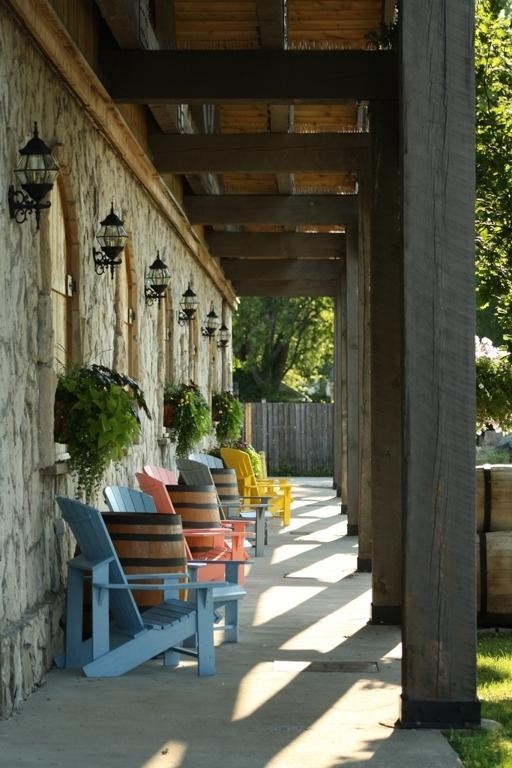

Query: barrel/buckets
[[475, 531, 512, 613], [61, 510, 190, 642], [167, 482, 223, 561], [474, 467, 512, 531], [178, 464, 241, 519]]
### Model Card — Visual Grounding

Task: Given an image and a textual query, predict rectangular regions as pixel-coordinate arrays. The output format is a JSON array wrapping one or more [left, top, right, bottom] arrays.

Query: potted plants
[[46, 358, 246, 507]]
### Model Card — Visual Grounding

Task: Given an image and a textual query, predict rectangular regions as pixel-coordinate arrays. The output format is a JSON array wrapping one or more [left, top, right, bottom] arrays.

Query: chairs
[[102, 484, 254, 644], [134, 447, 294, 589], [55, 494, 229, 679]]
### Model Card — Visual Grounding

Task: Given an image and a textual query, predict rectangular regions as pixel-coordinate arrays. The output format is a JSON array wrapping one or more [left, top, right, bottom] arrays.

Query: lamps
[[144, 250, 170, 306], [177, 281, 198, 325], [201, 308, 231, 348], [8, 121, 59, 232], [91, 200, 129, 280]]
